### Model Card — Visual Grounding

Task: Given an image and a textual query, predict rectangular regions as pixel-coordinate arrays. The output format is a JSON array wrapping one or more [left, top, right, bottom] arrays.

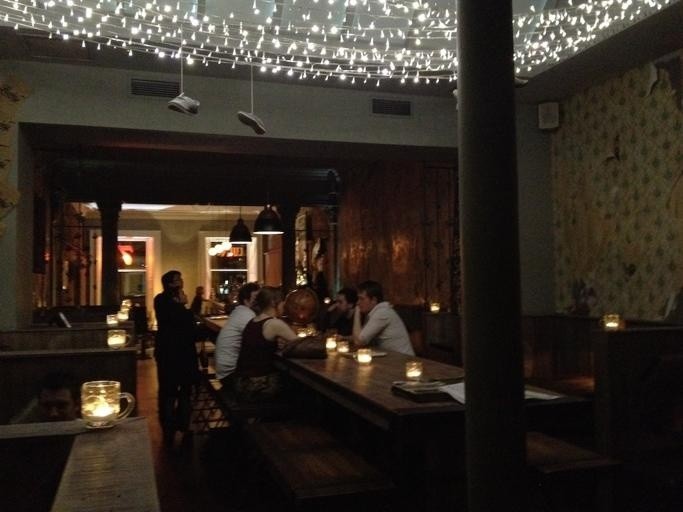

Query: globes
[[283, 285, 320, 331]]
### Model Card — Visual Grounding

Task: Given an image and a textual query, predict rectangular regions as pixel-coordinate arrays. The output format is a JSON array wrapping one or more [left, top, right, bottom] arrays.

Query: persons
[[153, 270, 204, 451], [214, 283, 263, 386], [231, 284, 299, 411], [190, 284, 224, 315], [320, 286, 367, 335], [352, 279, 416, 358], [36, 370, 82, 424], [163, 288, 203, 436]]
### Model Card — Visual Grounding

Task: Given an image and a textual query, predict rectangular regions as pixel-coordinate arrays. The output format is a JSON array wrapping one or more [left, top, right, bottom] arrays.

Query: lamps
[[252, 190, 285, 236], [229, 205, 252, 244]]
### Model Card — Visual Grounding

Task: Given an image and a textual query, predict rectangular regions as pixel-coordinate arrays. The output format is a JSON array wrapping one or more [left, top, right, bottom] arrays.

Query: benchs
[[195, 373, 385, 512], [524, 430, 621, 512]]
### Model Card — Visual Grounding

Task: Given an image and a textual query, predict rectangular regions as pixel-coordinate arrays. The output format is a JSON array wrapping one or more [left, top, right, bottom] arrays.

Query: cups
[[81, 381, 136, 430], [107, 330, 131, 349], [325, 337, 337, 350], [337, 338, 350, 353], [356, 348, 374, 364], [405, 360, 424, 380], [106, 297, 134, 328], [297, 326, 316, 338]]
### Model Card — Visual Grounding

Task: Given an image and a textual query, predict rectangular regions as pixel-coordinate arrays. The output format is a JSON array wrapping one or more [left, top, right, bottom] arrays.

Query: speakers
[[537, 101, 561, 130]]
